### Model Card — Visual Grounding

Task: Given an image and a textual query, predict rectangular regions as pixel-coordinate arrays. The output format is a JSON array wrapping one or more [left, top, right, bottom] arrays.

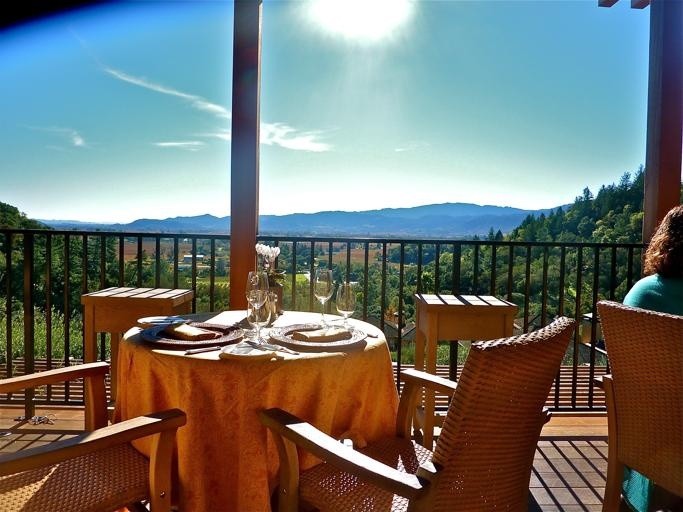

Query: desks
[[412, 293, 520, 452], [81, 285, 193, 425]]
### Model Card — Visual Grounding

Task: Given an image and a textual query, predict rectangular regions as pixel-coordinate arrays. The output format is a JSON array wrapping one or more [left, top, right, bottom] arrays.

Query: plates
[[137, 315, 185, 326], [268, 322, 368, 347], [141, 322, 246, 346]]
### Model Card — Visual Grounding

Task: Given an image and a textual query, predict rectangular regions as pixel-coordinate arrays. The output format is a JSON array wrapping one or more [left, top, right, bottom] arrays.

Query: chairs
[[0, 362, 188, 511], [259, 301, 575, 511], [590, 298, 683, 512]]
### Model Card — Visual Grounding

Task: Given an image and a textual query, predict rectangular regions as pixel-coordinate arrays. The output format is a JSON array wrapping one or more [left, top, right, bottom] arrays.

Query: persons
[[621, 205, 683, 512]]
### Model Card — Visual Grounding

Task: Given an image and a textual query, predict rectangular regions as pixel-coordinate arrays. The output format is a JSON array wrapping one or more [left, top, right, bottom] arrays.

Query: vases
[[259, 268, 284, 316]]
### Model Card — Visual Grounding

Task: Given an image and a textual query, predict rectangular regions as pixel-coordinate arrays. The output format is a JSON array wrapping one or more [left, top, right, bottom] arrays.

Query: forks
[[258, 337, 300, 355]]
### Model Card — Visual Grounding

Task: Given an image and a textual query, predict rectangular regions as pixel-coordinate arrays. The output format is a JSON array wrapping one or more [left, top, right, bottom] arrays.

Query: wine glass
[[245, 271, 271, 347], [336, 283, 357, 333], [312, 268, 334, 325]]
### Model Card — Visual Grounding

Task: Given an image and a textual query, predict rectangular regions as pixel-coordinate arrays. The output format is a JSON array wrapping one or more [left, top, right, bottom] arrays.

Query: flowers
[[256, 243, 289, 290]]
